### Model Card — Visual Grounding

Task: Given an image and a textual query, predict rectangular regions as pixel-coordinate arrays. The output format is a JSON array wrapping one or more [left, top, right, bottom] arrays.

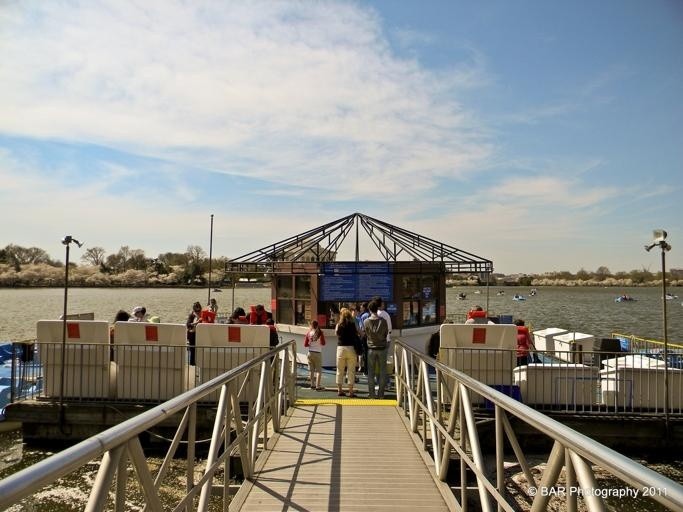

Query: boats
[[496, 290, 506, 296], [212, 287, 224, 292], [661, 294, 677, 300], [528, 288, 538, 296], [474, 289, 484, 294], [614, 295, 633, 303], [512, 295, 526, 300], [456, 291, 467, 300]]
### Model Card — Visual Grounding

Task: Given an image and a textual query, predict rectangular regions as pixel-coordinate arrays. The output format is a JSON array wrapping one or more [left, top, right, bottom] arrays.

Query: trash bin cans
[[592, 337, 619, 368]]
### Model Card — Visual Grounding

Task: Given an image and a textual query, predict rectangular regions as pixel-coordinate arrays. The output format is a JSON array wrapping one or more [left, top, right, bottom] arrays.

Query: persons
[[226, 304, 279, 364], [465, 305, 495, 324], [304, 320, 325, 390], [514, 319, 537, 366], [429, 319, 454, 374], [187, 299, 218, 365], [334, 296, 392, 398], [109, 306, 160, 361]]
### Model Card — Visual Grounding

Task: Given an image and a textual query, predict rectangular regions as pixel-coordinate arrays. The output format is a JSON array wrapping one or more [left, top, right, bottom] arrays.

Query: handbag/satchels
[[354, 335, 364, 357]]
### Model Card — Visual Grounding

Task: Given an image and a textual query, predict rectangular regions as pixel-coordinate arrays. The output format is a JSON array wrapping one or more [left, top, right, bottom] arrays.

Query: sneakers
[[311, 384, 385, 399]]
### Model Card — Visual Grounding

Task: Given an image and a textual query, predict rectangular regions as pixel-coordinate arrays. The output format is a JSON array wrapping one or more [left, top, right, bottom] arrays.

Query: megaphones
[[665, 243, 671, 251], [66, 236, 72, 242], [652, 229, 664, 242], [644, 244, 656, 252], [62, 238, 71, 245], [74, 239, 84, 247]]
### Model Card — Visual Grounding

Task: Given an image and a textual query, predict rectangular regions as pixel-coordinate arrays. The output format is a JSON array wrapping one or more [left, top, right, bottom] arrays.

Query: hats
[[146, 317, 159, 323], [130, 307, 142, 315]]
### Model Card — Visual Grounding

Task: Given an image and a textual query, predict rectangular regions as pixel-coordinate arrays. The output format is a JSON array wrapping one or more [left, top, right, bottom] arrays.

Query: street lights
[[58, 236, 84, 432], [644, 230, 671, 413]]
